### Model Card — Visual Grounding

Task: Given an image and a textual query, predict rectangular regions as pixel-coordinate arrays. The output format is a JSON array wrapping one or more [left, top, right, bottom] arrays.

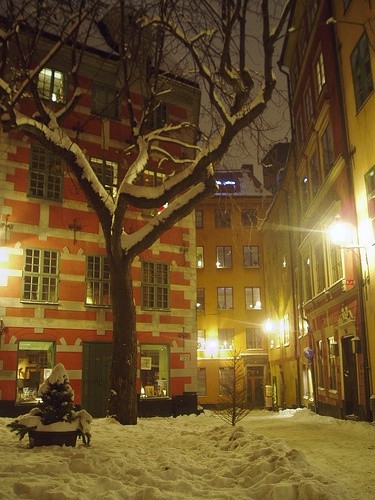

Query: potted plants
[[7, 363, 91, 447]]
